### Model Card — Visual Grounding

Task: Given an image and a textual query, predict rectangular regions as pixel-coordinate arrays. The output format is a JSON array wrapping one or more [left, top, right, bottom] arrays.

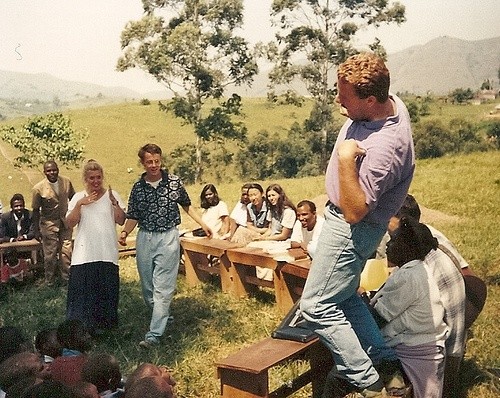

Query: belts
[[325, 199, 341, 213]]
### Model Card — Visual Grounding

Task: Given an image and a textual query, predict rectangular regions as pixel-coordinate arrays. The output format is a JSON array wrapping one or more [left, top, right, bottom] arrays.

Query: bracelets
[[113, 201, 118, 206], [121, 229, 128, 237]]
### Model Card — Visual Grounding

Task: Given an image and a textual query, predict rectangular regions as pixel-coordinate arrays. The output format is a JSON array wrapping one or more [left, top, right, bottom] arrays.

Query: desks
[[0, 239, 45, 267], [180, 237, 335, 398]]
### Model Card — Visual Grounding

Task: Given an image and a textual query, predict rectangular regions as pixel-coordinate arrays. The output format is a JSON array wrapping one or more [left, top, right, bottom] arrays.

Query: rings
[[88, 198, 91, 203]]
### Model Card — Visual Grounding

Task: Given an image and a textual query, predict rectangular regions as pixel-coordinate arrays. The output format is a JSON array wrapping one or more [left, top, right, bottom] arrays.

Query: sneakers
[[139, 340, 158, 348], [343, 361, 409, 398]]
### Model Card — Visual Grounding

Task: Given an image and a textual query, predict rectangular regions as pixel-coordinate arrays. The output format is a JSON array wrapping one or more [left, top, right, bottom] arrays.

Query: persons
[[200, 184, 229, 240], [229, 184, 251, 239], [58, 319, 91, 356], [253, 185, 297, 282], [36, 327, 63, 364], [119, 144, 212, 349], [387, 194, 479, 362], [300, 53, 415, 398], [1, 352, 175, 398], [1, 326, 30, 358], [66, 159, 125, 335], [1, 247, 43, 296], [32, 161, 76, 286], [246, 184, 271, 235], [323, 216, 450, 398], [291, 200, 325, 260], [0, 193, 37, 243]]
